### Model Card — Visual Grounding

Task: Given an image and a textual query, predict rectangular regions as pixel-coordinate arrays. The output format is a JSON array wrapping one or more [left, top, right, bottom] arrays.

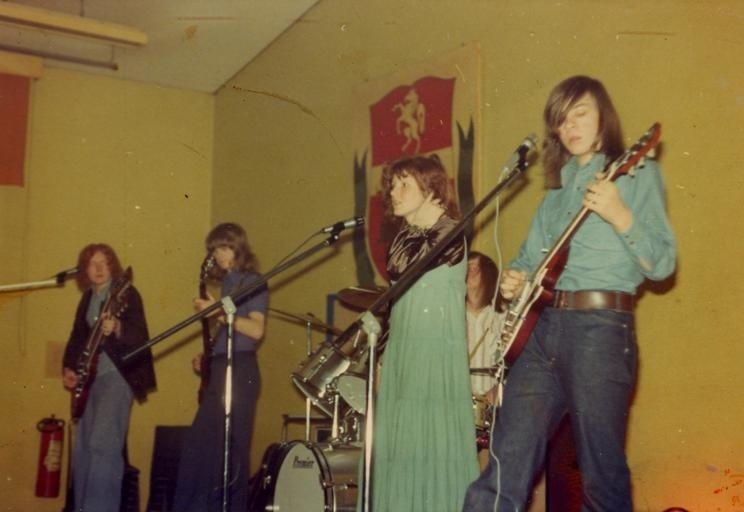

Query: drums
[[336, 358, 374, 415], [263, 439, 361, 512], [292, 342, 363, 428], [471, 394, 490, 432]]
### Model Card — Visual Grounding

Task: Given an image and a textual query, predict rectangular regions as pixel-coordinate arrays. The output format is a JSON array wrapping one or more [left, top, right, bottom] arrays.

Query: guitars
[[198, 256, 213, 402], [493, 122, 661, 372], [70, 265, 134, 420]]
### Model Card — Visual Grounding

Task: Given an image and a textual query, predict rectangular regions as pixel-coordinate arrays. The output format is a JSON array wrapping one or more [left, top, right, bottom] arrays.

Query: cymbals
[[339, 285, 391, 313]]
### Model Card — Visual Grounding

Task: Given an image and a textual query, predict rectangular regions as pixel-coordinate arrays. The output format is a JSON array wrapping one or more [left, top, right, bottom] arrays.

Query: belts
[[551, 289, 636, 312]]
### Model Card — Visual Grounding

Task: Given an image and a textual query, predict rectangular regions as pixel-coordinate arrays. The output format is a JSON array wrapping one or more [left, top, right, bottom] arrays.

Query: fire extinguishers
[[36, 414, 64, 498]]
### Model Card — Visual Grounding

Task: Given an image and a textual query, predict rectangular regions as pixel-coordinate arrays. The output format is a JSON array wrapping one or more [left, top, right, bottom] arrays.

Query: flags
[[370, 75, 456, 166]]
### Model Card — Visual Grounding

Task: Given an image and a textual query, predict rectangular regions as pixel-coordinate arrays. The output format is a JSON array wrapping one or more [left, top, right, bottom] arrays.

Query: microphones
[[57, 266, 84, 278], [502, 133, 539, 173], [321, 215, 364, 234]]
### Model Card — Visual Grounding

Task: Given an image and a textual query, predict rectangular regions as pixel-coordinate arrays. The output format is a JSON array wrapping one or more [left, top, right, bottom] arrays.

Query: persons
[[465, 251, 514, 437], [174, 223, 269, 512], [461, 75, 677, 512], [62, 244, 158, 512], [355, 156, 482, 512]]
